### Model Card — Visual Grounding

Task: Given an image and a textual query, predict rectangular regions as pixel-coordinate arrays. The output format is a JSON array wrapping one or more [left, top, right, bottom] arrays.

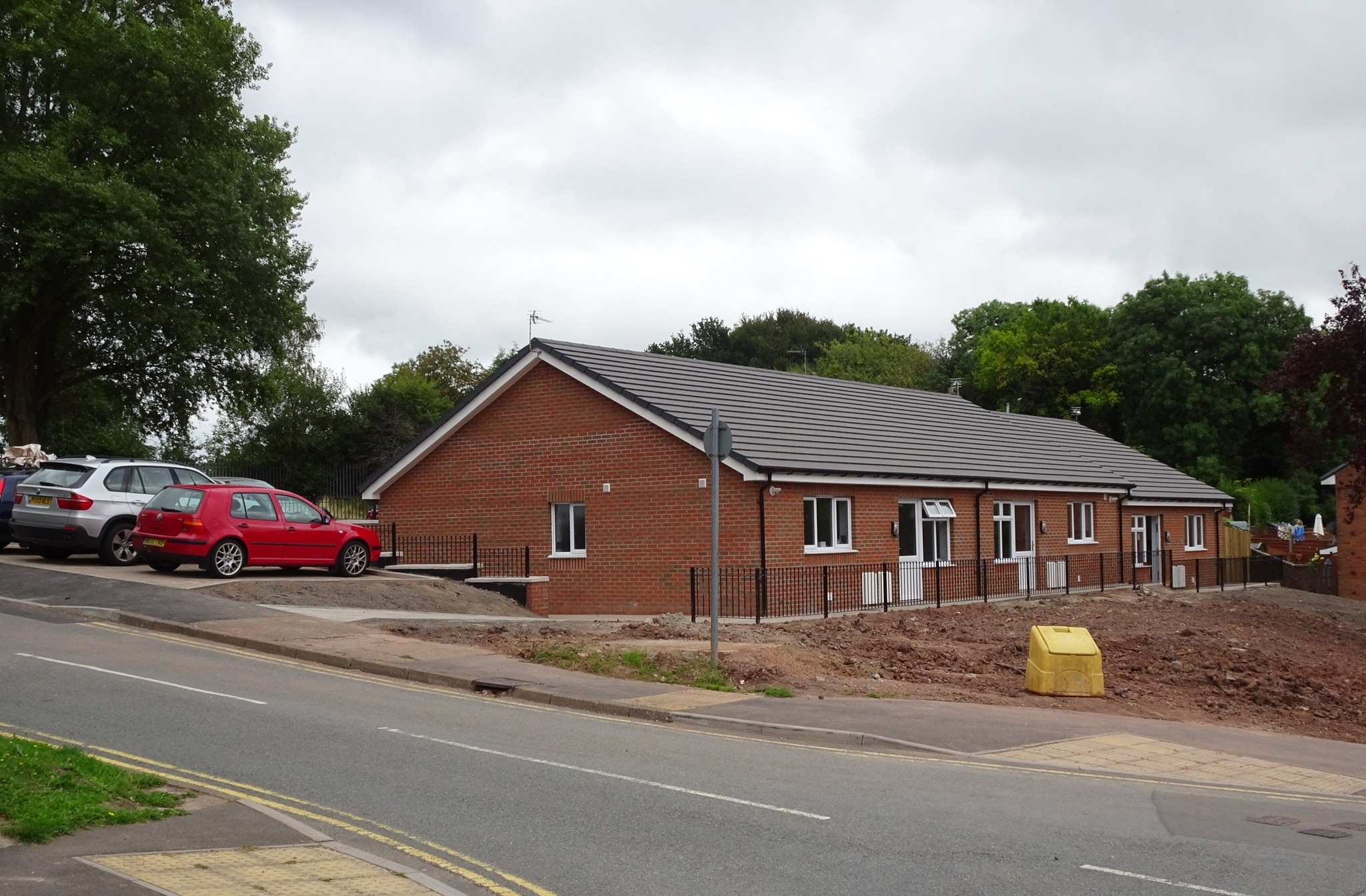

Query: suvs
[[7, 455, 261, 567]]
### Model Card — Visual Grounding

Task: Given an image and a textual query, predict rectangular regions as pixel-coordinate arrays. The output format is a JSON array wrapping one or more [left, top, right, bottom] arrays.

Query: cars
[[0, 467, 39, 552], [183, 475, 280, 501], [130, 483, 383, 580]]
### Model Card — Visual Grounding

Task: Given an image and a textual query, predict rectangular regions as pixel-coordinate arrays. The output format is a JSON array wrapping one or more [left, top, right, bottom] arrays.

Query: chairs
[[231, 498, 242, 511]]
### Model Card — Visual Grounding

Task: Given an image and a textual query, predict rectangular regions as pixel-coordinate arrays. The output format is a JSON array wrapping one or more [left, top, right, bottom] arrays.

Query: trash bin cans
[[1025, 625, 1105, 698]]
